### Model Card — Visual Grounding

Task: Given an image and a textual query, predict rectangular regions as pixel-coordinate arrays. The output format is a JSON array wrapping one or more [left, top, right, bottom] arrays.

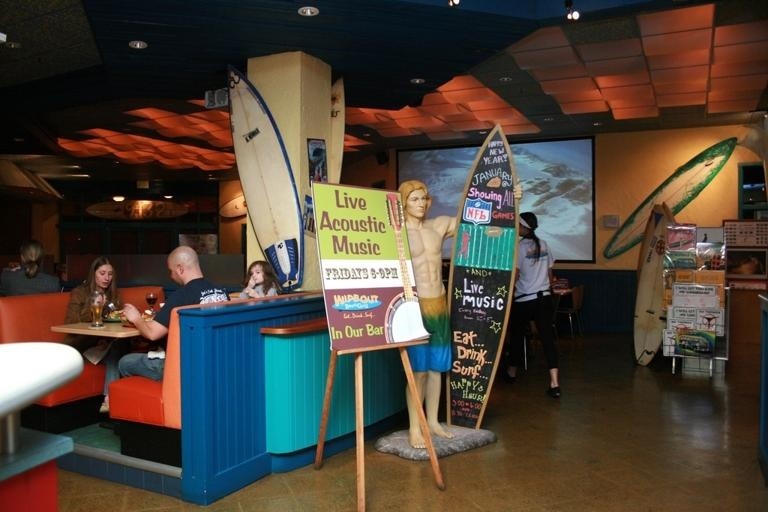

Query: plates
[[102, 318, 122, 322]]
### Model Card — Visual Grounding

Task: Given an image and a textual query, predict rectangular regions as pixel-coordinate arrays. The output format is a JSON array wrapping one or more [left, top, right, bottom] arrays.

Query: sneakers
[[545, 384, 561, 398], [498, 369, 518, 384], [99, 403, 110, 413]]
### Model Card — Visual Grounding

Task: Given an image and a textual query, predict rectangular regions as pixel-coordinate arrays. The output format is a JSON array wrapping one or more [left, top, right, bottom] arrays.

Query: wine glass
[[146, 293, 158, 320]]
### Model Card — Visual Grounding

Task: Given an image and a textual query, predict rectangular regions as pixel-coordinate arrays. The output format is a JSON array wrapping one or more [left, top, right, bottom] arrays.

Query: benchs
[[109, 292, 315, 470], [0, 287, 166, 433]]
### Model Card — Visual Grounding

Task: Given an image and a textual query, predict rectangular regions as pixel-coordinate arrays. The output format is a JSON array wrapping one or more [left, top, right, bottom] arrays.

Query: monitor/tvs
[[396, 135, 595, 263]]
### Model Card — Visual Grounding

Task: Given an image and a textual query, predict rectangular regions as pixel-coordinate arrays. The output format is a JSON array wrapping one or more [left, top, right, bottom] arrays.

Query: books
[[663, 223, 727, 358]]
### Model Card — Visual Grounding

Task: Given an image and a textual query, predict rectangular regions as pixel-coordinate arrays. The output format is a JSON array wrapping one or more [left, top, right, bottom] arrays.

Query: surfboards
[[634, 204, 674, 365], [603, 138, 737, 258], [219, 196, 247, 218], [228, 68, 305, 292]]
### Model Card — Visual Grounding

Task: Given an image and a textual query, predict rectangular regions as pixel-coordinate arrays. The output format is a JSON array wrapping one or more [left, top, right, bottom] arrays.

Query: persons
[[503, 212, 562, 398], [0, 238, 63, 297], [118, 246, 231, 380], [240, 260, 277, 298], [64, 254, 121, 413], [398, 179, 522, 450]]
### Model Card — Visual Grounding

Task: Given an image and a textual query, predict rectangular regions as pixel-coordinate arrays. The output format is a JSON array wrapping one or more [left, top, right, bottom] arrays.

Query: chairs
[[553, 282, 586, 343]]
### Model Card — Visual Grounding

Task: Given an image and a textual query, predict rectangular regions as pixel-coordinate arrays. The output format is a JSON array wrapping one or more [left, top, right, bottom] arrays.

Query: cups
[[89, 290, 105, 329]]
